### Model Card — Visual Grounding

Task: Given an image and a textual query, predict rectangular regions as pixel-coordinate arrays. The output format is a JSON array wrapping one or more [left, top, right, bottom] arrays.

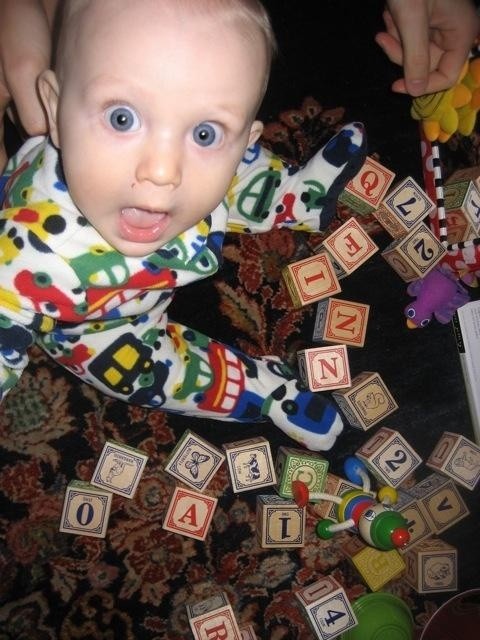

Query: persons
[[0, 0, 369, 454], [292, 458, 410, 553], [0, 0, 480, 176]]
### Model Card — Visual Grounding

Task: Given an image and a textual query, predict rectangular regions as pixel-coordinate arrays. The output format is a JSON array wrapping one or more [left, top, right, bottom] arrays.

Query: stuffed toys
[[411, 54, 480, 146], [405, 271, 470, 328]]
